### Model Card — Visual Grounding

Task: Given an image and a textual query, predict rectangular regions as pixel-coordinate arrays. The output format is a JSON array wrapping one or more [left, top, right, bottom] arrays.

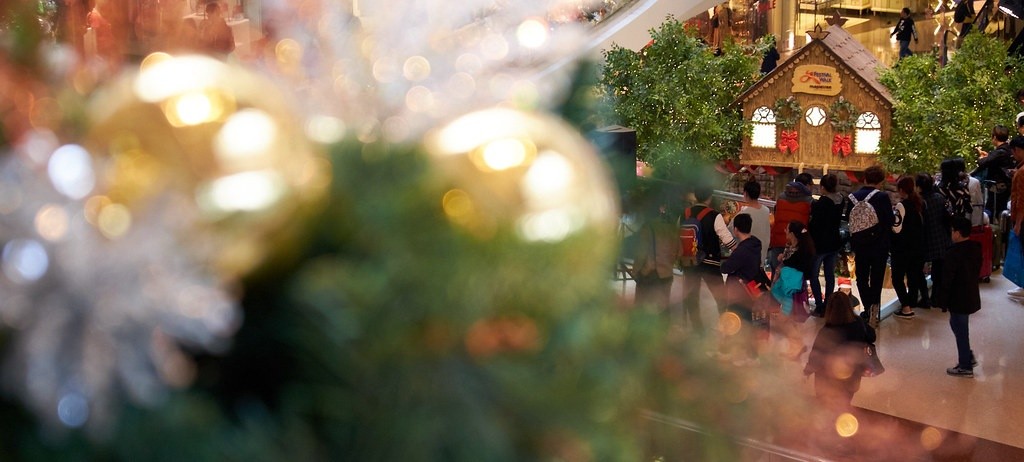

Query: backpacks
[[677, 207, 714, 269], [847, 190, 878, 234]]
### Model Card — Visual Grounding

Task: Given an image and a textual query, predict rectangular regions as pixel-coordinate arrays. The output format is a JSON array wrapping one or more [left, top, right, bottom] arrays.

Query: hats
[[837, 276, 852, 289]]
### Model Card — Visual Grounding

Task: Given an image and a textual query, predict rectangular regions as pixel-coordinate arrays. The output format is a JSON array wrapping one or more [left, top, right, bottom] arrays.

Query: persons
[[889, 8, 919, 68], [956, 0, 995, 38], [634, 112, 1024, 368], [802, 292, 875, 404], [930, 216, 982, 378], [713, 2, 734, 54], [760, 39, 780, 75]]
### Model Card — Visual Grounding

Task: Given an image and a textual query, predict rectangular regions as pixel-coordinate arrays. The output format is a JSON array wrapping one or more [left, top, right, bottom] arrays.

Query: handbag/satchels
[[638, 270, 661, 286], [861, 325, 885, 377], [793, 290, 812, 322]]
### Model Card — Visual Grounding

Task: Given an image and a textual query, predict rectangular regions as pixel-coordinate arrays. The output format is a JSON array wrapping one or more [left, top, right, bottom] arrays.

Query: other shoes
[[1006, 286, 1024, 299], [971, 350, 979, 367], [946, 364, 973, 377], [917, 298, 931, 309], [868, 303, 878, 329], [979, 277, 991, 283], [706, 349, 732, 362], [733, 357, 761, 367], [893, 309, 915, 319], [790, 344, 808, 362]]
